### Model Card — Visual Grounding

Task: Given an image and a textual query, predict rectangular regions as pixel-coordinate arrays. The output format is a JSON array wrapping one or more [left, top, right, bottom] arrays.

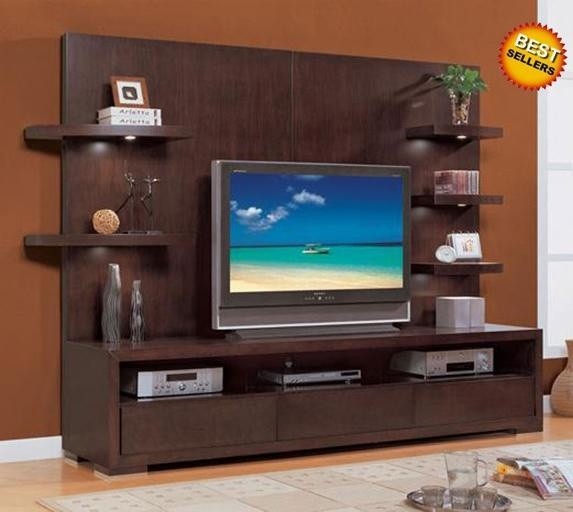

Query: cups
[[447, 487, 473, 509], [420, 484, 446, 508], [443, 449, 489, 497], [473, 487, 497, 509]]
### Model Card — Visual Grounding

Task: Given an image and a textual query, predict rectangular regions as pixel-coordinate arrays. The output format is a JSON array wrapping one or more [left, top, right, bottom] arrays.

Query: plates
[[406, 488, 512, 512]]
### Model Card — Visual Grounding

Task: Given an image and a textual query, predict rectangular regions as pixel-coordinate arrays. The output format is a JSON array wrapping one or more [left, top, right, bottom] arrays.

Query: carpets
[[32, 435, 573, 512]]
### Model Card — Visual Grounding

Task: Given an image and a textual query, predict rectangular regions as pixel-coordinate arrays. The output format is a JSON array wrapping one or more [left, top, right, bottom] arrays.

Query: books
[[491, 456, 573, 501], [433, 169, 480, 207], [446, 231, 483, 261]]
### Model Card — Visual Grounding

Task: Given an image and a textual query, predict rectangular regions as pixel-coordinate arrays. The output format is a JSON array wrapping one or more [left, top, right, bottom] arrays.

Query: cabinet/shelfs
[[19, 31, 545, 479]]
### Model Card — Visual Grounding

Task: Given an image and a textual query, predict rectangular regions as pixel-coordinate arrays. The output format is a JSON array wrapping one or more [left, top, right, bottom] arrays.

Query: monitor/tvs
[[211, 159, 413, 340]]
